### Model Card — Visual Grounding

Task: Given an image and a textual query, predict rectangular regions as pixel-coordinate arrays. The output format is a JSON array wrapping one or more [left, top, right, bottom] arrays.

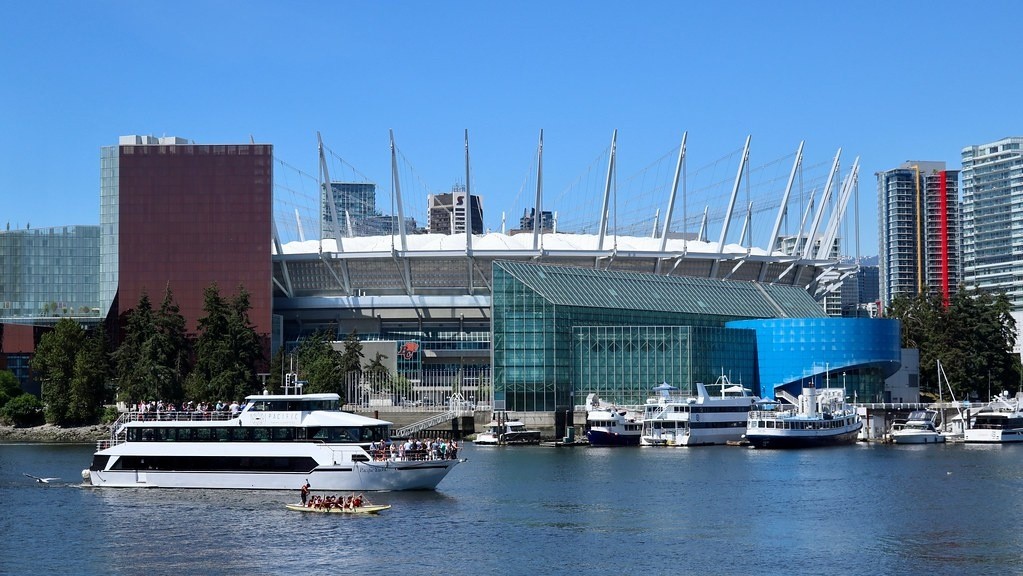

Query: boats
[[284, 497, 392, 514], [639, 366, 767, 447], [473, 413, 543, 444], [745, 362, 864, 447], [964, 390, 1023, 444], [586, 408, 641, 449], [77, 389, 468, 494], [889, 359, 964, 444]]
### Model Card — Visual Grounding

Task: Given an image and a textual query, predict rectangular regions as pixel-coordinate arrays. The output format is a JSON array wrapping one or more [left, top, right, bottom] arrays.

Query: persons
[[301, 484, 310, 506], [308, 494, 363, 509], [370, 437, 460, 462], [610, 403, 615, 412], [137, 399, 257, 421]]
